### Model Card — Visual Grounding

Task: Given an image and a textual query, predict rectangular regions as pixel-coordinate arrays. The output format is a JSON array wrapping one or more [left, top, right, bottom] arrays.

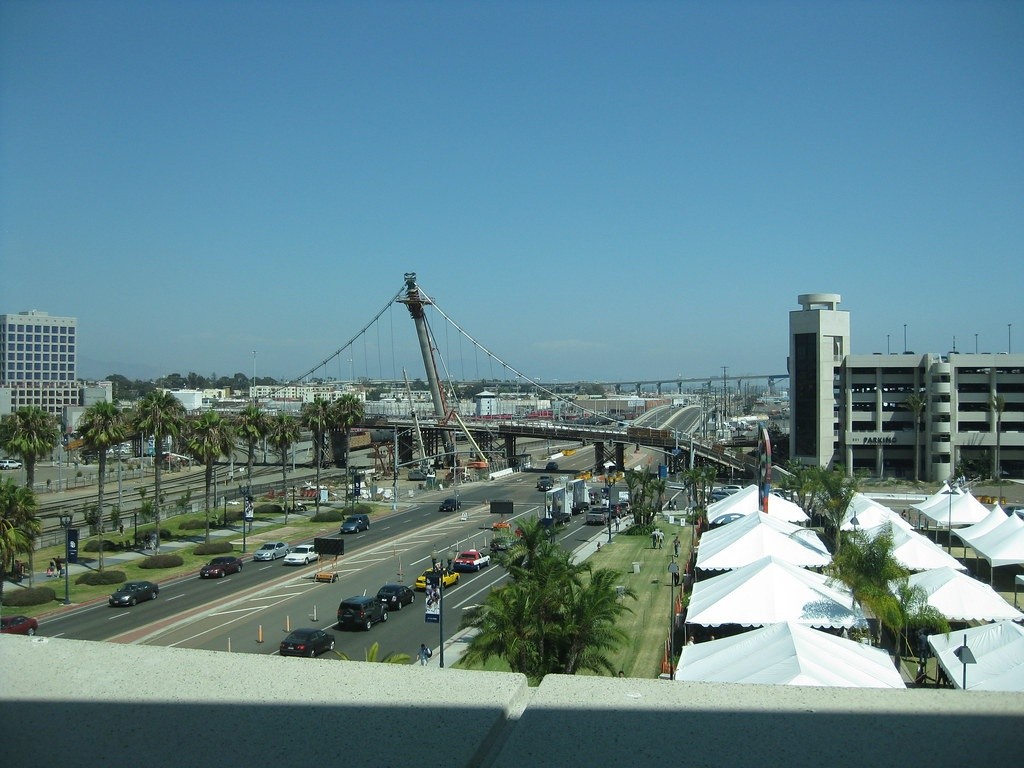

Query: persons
[[13, 559, 25, 582], [618, 671, 624, 678], [901, 509, 906, 519], [710, 635, 715, 641], [653, 530, 664, 549], [46, 555, 65, 579], [296, 501, 308, 511], [687, 633, 695, 645], [615, 515, 621, 531], [673, 564, 680, 587], [673, 536, 680, 558], [119, 524, 124, 536], [917, 630, 928, 666], [47, 478, 52, 488], [668, 497, 677, 510], [511, 458, 520, 474], [416, 643, 432, 666], [143, 531, 157, 551], [957, 474, 965, 487]]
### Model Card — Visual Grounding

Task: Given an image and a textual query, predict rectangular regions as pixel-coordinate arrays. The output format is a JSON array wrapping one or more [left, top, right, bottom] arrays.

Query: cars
[[708, 484, 785, 503], [415, 568, 460, 591], [537, 475, 554, 491], [546, 462, 558, 470], [254, 543, 321, 565], [572, 502, 631, 525], [0, 615, 38, 636], [200, 556, 243, 578], [490, 513, 570, 550], [453, 551, 490, 572], [439, 499, 461, 512], [280, 628, 335, 658], [109, 581, 159, 607]]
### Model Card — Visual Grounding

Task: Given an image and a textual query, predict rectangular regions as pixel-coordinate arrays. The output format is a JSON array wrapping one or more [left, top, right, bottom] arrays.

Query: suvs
[[0, 460, 22, 470], [337, 584, 415, 631]]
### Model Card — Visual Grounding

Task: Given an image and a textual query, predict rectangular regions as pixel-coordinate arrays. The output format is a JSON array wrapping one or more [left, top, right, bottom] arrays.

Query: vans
[[340, 466, 370, 533]]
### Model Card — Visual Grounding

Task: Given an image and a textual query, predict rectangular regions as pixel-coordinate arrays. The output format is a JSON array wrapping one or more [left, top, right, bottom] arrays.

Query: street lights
[[238, 481, 251, 553], [57, 507, 74, 604], [133, 507, 138, 544], [605, 474, 616, 543], [431, 544, 454, 667]]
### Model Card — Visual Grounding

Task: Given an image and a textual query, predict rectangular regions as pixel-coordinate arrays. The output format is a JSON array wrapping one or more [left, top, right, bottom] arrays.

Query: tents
[[676, 481, 1024, 690]]
